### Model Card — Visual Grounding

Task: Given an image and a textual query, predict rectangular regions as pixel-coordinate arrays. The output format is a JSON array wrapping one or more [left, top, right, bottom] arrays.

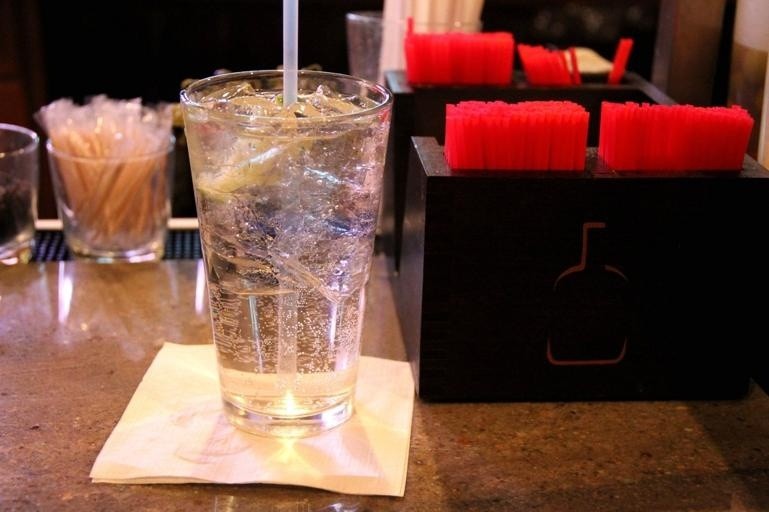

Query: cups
[[346, 10, 483, 82], [1, 119, 178, 267], [178, 68, 394, 442]]
